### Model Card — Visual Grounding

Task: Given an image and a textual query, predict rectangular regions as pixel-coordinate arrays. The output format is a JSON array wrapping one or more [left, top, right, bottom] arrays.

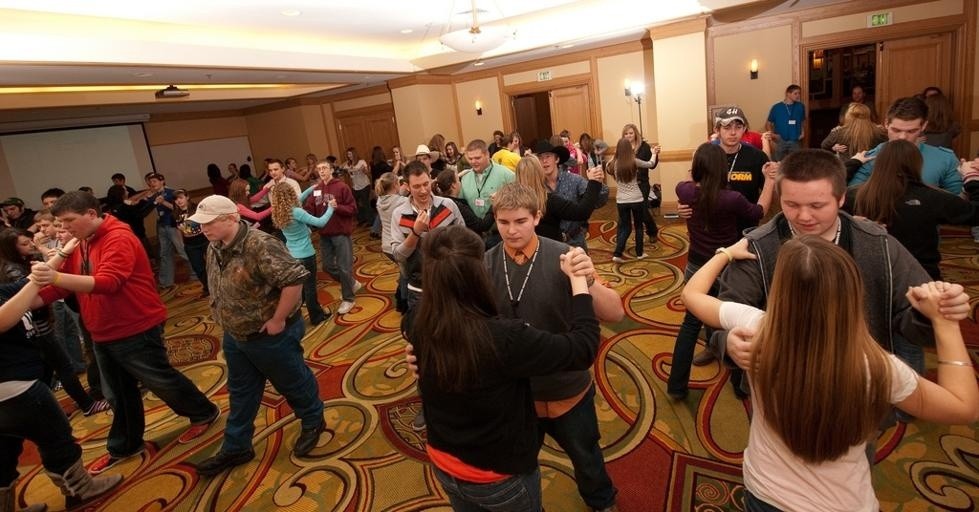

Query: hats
[[713, 105, 747, 128], [406, 144, 441, 163], [529, 137, 570, 165], [183, 193, 240, 225]]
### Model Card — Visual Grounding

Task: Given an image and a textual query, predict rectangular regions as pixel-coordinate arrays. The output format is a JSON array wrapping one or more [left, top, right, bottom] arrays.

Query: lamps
[[437, 1, 516, 60], [475, 100, 482, 115], [624, 80, 632, 96], [750, 59, 759, 80]]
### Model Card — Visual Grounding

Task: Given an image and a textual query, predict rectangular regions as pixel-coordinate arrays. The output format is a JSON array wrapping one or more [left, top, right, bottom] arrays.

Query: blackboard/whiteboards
[[150, 135, 258, 192]]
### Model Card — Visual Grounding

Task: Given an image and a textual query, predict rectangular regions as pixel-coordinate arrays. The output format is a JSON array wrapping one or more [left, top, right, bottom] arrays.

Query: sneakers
[[88, 447, 144, 477], [312, 312, 333, 327], [81, 402, 113, 415], [667, 390, 688, 400], [612, 255, 627, 263], [649, 236, 657, 244], [293, 421, 327, 459], [194, 447, 256, 477], [636, 253, 649, 259], [692, 344, 715, 367], [199, 288, 210, 298], [336, 300, 356, 315], [353, 279, 363, 295], [368, 232, 382, 241], [176, 409, 223, 444]]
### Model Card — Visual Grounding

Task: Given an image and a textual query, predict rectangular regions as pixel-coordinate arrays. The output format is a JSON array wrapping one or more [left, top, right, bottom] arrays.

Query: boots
[[45, 451, 123, 512], [0, 479, 48, 512]]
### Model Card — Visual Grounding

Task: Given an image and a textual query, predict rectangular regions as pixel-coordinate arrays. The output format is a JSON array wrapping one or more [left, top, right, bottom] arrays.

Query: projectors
[[155, 90, 190, 98]]
